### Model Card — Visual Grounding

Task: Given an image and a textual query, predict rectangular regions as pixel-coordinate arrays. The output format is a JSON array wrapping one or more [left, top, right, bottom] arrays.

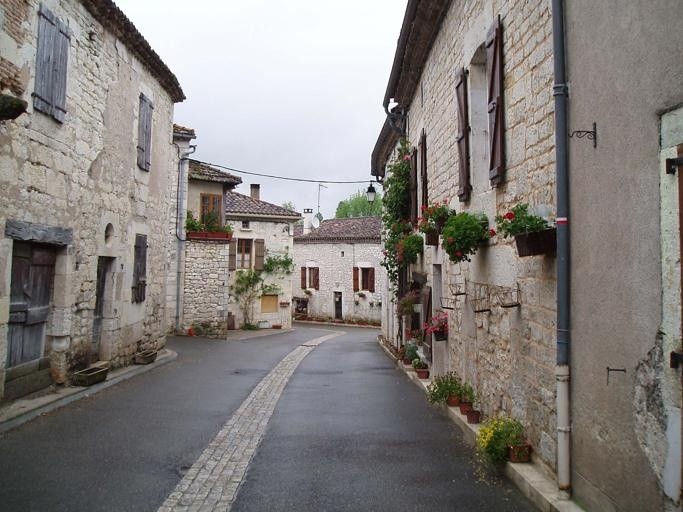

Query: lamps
[[367, 179, 382, 204]]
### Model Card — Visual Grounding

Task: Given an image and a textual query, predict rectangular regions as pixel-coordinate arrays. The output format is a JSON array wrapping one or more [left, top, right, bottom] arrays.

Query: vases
[[514, 228, 557, 257], [434, 331, 446, 341], [459, 402, 472, 414], [467, 410, 481, 423], [510, 442, 531, 461]]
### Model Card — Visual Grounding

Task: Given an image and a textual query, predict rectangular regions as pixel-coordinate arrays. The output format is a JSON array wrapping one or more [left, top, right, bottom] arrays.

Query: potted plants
[[412, 358, 429, 379], [185, 209, 235, 238], [426, 371, 475, 407]]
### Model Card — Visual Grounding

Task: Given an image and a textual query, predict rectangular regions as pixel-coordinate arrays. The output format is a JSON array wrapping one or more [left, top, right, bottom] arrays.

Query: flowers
[[379, 141, 496, 265], [421, 307, 449, 336], [396, 288, 420, 315], [496, 200, 549, 238], [474, 414, 525, 466], [404, 327, 422, 338]]
